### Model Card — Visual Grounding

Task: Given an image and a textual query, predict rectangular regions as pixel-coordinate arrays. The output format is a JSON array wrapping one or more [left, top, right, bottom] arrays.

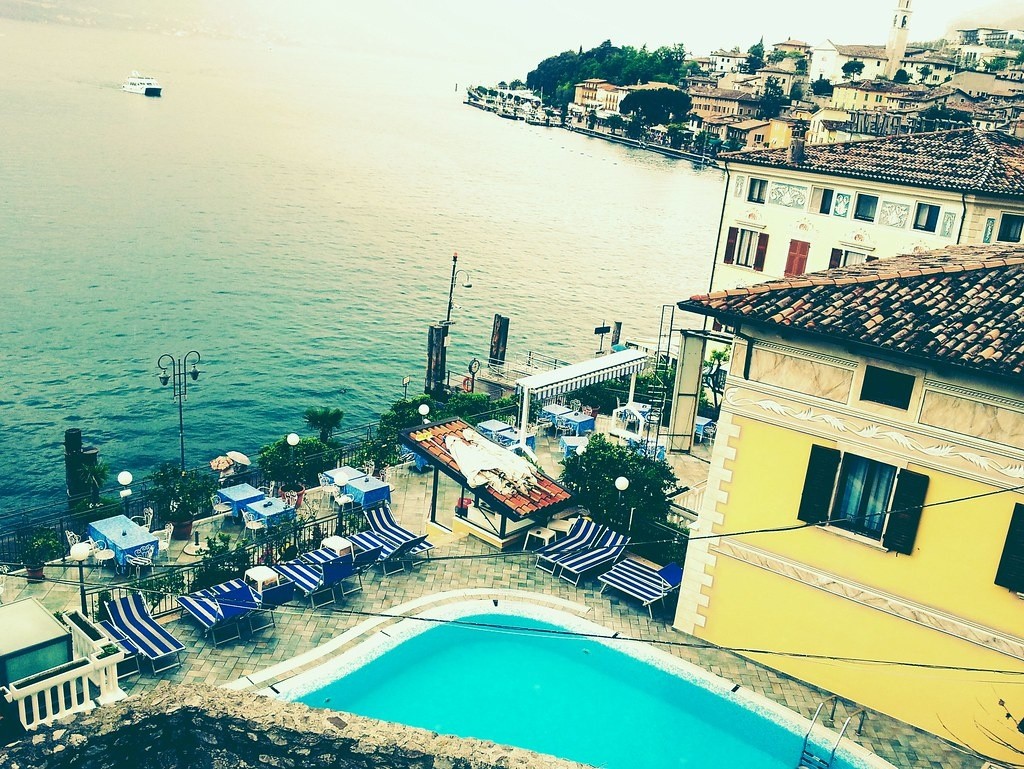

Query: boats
[[119, 70, 164, 97]]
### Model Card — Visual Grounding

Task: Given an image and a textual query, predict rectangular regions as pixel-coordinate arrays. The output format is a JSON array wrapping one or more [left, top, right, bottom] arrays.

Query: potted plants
[[259, 433, 336, 511], [15, 525, 67, 581], [144, 462, 218, 538], [584, 390, 601, 420], [351, 437, 398, 482]]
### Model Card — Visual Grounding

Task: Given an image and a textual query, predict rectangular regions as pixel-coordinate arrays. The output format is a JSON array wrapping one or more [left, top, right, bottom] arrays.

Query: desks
[[494, 427, 536, 454], [217, 483, 264, 524], [245, 496, 295, 538], [559, 436, 590, 458], [556, 410, 595, 437], [86, 515, 160, 576], [695, 415, 713, 441], [541, 403, 573, 428], [622, 401, 651, 432], [323, 465, 367, 506], [244, 565, 279, 595], [343, 475, 392, 514], [521, 526, 557, 551], [319, 534, 355, 561], [543, 518, 573, 537], [476, 420, 512, 437]]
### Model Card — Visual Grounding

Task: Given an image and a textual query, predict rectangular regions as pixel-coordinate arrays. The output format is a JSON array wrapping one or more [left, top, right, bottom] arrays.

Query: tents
[[650, 123, 668, 136]]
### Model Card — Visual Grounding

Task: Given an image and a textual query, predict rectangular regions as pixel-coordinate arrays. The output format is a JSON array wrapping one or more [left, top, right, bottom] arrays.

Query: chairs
[[476, 415, 536, 448], [131, 506, 153, 532], [300, 545, 383, 598], [557, 530, 631, 587], [535, 399, 593, 441], [175, 586, 249, 649], [208, 578, 295, 636], [365, 504, 434, 568], [271, 552, 354, 610], [239, 509, 265, 541], [702, 419, 718, 445], [531, 517, 601, 575], [209, 495, 234, 525], [104, 591, 186, 673], [615, 396, 665, 434], [346, 529, 429, 581], [316, 459, 396, 514], [597, 558, 683, 620], [158, 523, 174, 565], [64, 529, 115, 578], [91, 619, 141, 682]]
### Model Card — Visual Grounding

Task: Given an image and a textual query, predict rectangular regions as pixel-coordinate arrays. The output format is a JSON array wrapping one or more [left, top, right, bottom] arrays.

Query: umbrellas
[[226, 451, 251, 466], [210, 456, 234, 470], [444, 429, 555, 502]]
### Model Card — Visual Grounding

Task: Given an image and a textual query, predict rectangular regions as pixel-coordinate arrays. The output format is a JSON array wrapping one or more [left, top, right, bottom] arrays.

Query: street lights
[[287, 432, 300, 482], [116, 470, 133, 519], [70, 540, 91, 618], [156, 349, 202, 480], [614, 475, 630, 532], [417, 404, 430, 425], [445, 250, 472, 324]]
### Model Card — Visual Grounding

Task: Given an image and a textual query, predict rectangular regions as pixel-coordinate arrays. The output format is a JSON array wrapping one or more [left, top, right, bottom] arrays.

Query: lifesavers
[[462, 377, 472, 392]]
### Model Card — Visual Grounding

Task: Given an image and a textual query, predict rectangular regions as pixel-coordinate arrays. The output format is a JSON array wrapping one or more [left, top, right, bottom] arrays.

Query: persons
[[220, 464, 247, 486]]
